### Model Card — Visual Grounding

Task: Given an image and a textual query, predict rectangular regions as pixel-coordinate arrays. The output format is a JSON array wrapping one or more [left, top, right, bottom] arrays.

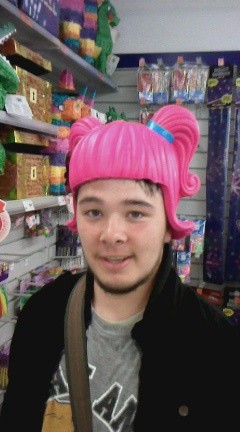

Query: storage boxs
[[1, 40, 55, 123], [1, 129, 51, 200]]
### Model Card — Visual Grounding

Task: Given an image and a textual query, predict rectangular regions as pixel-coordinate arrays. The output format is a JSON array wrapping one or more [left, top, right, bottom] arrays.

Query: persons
[[2, 105, 240, 432]]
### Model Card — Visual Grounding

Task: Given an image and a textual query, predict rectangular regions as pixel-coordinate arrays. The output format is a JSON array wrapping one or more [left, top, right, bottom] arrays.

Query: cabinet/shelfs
[[0, 0, 118, 217]]
